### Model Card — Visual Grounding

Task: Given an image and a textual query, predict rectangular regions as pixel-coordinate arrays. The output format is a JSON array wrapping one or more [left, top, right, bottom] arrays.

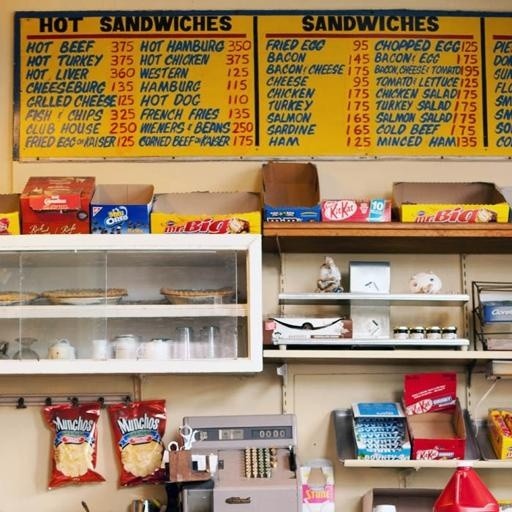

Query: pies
[[161, 287, 236, 297], [0, 290, 39, 303], [41, 288, 128, 297]]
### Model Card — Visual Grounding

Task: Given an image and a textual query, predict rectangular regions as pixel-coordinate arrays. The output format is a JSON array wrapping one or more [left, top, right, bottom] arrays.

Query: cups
[[373, 505, 397, 512], [132, 499, 156, 512], [94, 325, 245, 361]]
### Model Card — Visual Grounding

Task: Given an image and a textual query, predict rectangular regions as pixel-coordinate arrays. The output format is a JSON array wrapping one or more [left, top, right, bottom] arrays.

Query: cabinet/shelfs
[[263, 221, 511, 470], [0, 234, 264, 376]]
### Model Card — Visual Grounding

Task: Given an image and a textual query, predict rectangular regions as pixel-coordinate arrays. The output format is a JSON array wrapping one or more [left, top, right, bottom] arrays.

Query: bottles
[[48, 337, 76, 359]]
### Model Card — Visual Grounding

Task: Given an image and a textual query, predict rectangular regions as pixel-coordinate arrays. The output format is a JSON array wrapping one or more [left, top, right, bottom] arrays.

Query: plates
[[1, 298, 40, 307], [159, 292, 239, 303], [43, 294, 130, 306]]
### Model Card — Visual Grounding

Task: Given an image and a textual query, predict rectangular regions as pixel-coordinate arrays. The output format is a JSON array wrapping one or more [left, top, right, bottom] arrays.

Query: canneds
[[393, 326, 457, 340]]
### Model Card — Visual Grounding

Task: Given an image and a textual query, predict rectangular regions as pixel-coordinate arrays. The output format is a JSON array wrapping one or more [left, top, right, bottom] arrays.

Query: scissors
[[168, 441, 189, 452], [180, 424, 204, 450]]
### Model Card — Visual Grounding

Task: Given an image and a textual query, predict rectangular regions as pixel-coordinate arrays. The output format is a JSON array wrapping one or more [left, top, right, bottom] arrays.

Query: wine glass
[[13, 337, 40, 360]]
